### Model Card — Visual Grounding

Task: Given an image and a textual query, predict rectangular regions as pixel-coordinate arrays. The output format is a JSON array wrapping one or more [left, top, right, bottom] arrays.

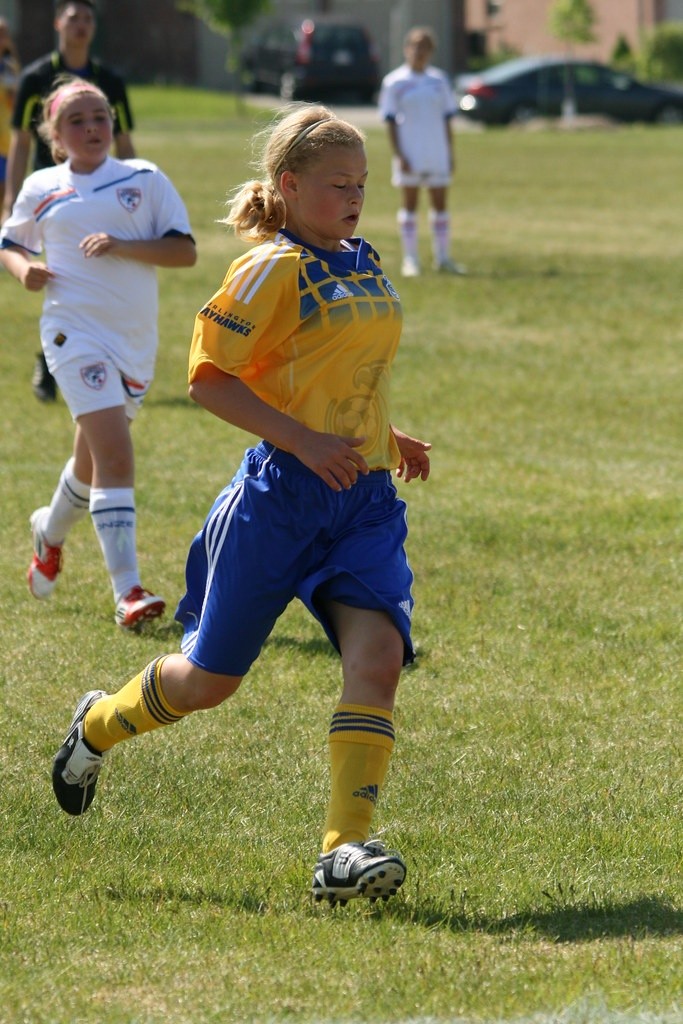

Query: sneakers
[[311, 829, 407, 908], [114, 584, 166, 630], [51, 689, 113, 817], [32, 351, 57, 404], [26, 506, 64, 601]]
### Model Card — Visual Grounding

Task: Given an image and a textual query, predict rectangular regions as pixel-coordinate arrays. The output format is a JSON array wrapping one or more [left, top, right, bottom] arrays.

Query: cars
[[454, 54, 682, 133]]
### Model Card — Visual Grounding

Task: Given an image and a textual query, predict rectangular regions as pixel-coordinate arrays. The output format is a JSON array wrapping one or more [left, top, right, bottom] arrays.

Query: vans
[[239, 18, 378, 102]]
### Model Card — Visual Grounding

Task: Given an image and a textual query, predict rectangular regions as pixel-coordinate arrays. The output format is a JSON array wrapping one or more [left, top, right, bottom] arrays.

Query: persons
[[52, 108, 435, 901], [0, 19, 23, 213], [1, 0, 138, 398], [0, 79, 202, 627], [376, 28, 471, 281]]
